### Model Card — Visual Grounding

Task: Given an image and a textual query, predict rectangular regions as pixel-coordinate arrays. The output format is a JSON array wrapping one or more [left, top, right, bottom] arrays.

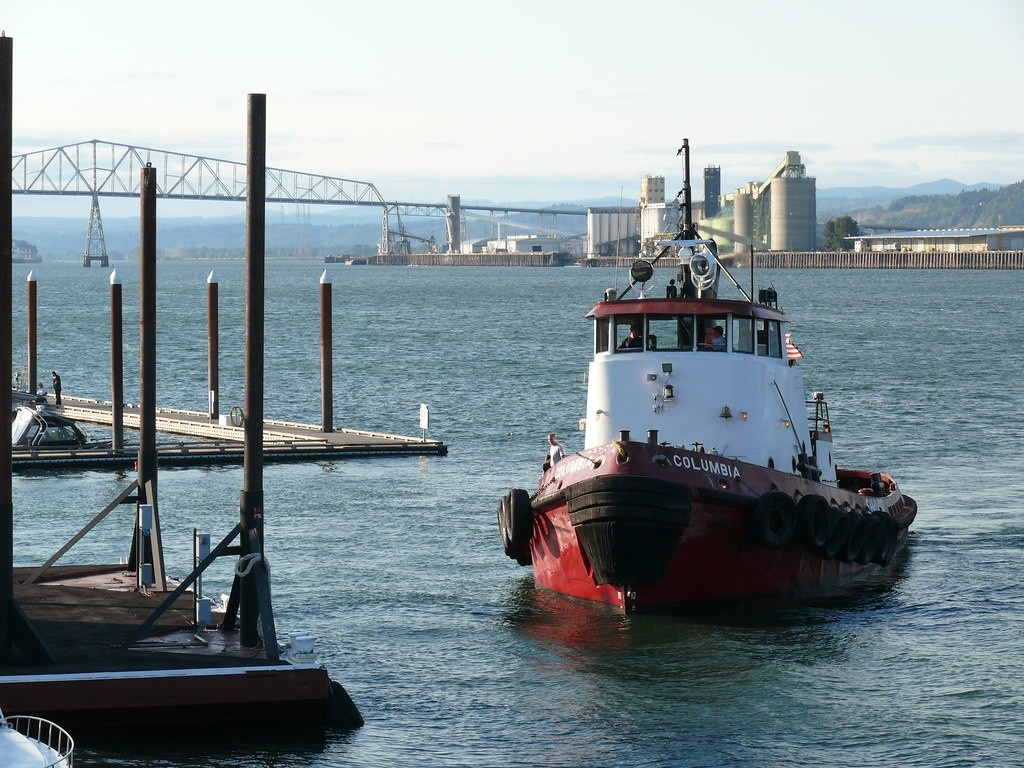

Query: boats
[[12, 388, 48, 416], [497, 138, 919, 621], [12, 406, 129, 449]]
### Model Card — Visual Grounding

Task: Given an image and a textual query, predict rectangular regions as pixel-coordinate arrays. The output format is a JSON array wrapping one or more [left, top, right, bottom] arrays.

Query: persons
[[52, 371, 61, 405], [712, 326, 726, 351], [617, 323, 644, 348], [548, 434, 565, 467], [37, 383, 47, 400]]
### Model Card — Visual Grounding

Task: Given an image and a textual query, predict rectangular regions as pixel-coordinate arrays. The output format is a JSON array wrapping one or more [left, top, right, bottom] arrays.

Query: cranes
[[395, 200, 411, 254]]
